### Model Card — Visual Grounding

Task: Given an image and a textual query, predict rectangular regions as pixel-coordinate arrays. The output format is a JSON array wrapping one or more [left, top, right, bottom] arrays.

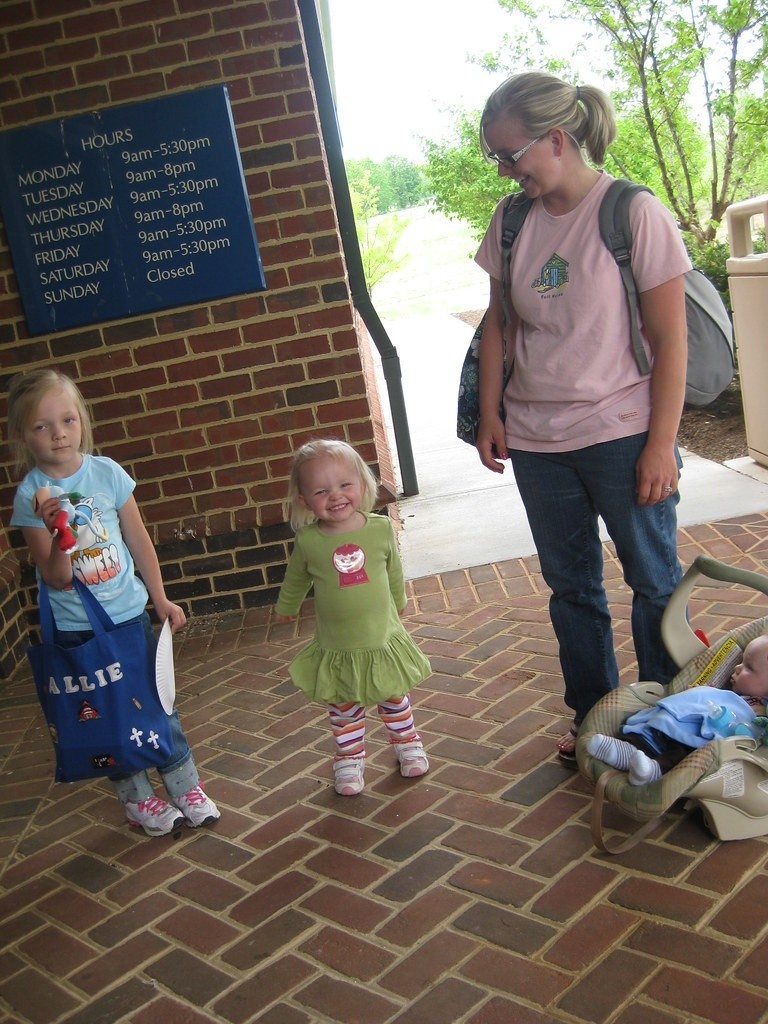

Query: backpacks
[[500, 177, 736, 407]]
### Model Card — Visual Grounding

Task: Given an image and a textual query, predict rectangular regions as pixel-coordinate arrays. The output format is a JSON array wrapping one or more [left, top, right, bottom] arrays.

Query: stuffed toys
[[32, 485, 108, 550]]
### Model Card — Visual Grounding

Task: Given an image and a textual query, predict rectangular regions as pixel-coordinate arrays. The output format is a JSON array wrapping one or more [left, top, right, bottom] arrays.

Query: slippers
[[558, 728, 577, 761]]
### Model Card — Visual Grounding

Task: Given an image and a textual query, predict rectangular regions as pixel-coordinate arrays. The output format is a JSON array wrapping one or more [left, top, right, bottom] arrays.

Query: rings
[[663, 487, 672, 492]]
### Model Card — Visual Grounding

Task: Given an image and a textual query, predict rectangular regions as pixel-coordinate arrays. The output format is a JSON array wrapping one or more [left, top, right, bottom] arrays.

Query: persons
[[274, 440, 431, 795], [10, 369, 221, 836], [587, 634, 768, 785], [473, 72, 693, 759]]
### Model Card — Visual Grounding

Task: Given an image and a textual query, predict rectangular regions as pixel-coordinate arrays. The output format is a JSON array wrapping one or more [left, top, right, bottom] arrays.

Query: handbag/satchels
[[24, 575, 176, 782], [457, 302, 512, 456]]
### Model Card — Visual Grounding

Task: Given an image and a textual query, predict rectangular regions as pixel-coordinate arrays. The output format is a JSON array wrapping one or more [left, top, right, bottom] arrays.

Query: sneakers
[[394, 735, 428, 777], [332, 757, 365, 795], [171, 785, 221, 827], [125, 795, 185, 836]]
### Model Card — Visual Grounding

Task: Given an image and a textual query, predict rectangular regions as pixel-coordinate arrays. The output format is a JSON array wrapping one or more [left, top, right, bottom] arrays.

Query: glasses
[[486, 131, 548, 168]]
